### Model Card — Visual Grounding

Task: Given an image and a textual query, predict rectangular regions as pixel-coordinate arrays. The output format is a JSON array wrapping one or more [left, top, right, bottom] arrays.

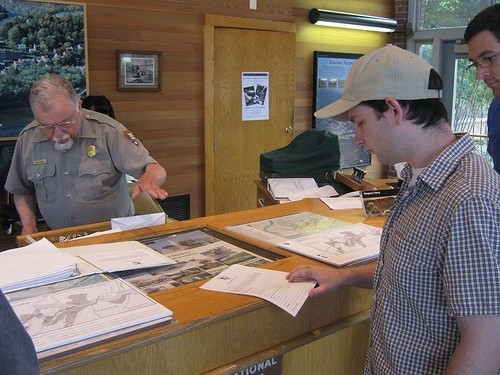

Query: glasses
[[37, 112, 75, 131], [466, 53, 500, 73]]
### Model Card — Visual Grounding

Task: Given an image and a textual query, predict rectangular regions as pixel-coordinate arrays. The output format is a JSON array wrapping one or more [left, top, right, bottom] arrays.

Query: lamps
[[309, 8, 398, 33]]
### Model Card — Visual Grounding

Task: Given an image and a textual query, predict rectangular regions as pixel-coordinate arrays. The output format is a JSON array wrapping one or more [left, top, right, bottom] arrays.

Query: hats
[[315, 44, 443, 122]]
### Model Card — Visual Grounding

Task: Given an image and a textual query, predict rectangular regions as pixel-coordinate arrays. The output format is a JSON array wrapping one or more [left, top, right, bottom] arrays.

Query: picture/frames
[[0, 0, 90, 141], [116, 50, 161, 91]]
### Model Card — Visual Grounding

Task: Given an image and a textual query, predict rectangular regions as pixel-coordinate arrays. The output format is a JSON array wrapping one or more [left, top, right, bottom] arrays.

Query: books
[[0, 238, 177, 365], [233, 213, 385, 269], [262, 177, 398, 216]]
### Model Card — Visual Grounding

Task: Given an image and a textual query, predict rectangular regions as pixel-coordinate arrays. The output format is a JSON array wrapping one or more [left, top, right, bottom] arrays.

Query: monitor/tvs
[[258, 127, 340, 186]]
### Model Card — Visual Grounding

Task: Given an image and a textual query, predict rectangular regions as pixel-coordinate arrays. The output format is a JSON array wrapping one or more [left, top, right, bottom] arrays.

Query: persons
[[286, 42, 500, 375], [5, 73, 167, 235], [464, 3, 500, 180]]
